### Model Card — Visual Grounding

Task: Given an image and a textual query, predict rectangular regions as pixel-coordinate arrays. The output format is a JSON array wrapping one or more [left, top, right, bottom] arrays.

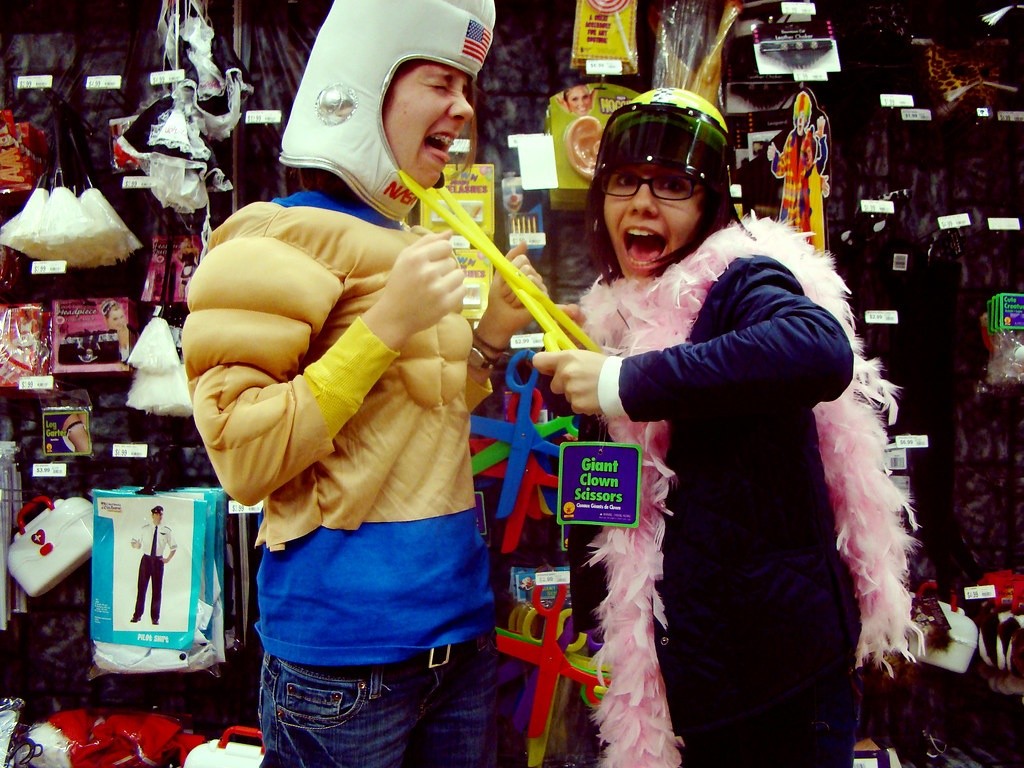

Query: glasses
[[599, 170, 705, 200]]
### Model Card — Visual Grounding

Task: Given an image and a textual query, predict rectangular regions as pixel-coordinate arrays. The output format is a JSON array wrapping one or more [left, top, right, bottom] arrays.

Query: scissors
[[399, 168, 603, 355], [467, 349, 579, 554], [490, 578, 615, 768]]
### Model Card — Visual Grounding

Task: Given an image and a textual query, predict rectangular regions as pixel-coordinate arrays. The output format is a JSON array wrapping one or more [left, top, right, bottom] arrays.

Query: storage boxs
[[48, 297, 140, 379], [901, 580, 978, 674], [183, 725, 266, 768]]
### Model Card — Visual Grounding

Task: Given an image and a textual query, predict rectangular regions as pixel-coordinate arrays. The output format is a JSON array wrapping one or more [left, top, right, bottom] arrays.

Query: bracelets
[[474, 329, 506, 353]]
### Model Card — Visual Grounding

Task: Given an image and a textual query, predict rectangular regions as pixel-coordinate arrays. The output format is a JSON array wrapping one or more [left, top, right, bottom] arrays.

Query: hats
[[151, 506, 164, 513], [278, 0, 496, 221]]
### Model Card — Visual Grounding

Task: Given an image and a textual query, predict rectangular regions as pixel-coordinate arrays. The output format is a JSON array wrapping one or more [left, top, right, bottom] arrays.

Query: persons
[[130, 506, 177, 625], [564, 83, 592, 114], [531, 88, 863, 768], [101, 298, 129, 362], [57, 413, 88, 452], [176, 236, 199, 300], [185, 52, 546, 768]]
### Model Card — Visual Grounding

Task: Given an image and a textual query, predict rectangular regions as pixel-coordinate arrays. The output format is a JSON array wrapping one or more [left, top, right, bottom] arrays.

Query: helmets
[[595, 87, 732, 201]]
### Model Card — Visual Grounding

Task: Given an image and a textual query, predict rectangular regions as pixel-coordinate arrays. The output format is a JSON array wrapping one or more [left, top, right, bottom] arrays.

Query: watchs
[[468, 345, 500, 370]]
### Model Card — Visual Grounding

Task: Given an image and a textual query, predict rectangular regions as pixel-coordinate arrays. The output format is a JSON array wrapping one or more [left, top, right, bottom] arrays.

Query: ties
[[151, 525, 158, 556]]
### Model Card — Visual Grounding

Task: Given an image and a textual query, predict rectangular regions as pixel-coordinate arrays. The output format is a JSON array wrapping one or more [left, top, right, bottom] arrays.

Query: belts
[[298, 638, 476, 683]]
